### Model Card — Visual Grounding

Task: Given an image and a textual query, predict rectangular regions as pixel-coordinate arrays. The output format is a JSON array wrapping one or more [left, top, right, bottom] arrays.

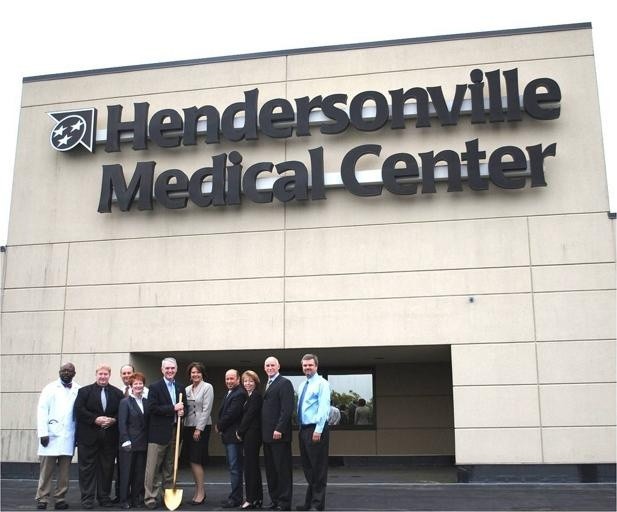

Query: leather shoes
[[296, 503, 310, 510], [311, 503, 324, 511], [223, 498, 241, 508], [264, 502, 276, 509], [54, 502, 68, 510], [37, 502, 47, 509]]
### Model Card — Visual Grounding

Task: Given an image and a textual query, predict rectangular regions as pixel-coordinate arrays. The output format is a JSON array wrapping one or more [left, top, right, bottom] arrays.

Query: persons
[[143, 357, 189, 509], [260, 356, 294, 511], [328, 399, 372, 425], [214, 369, 246, 508], [111, 363, 149, 504], [35, 362, 83, 509], [296, 353, 332, 511], [184, 362, 214, 505], [73, 364, 125, 509], [118, 373, 148, 509], [235, 370, 263, 510]]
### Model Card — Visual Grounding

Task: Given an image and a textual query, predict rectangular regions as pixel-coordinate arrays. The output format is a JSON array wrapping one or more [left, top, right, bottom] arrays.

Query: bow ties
[[62, 383, 72, 388], [169, 380, 176, 386]]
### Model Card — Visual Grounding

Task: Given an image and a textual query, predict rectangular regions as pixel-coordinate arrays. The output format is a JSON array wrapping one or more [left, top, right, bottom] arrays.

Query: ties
[[265, 380, 272, 390], [297, 380, 309, 426], [100, 388, 107, 413]]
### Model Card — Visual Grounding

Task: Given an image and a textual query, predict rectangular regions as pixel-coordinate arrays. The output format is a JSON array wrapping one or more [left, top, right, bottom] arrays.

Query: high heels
[[193, 493, 206, 505]]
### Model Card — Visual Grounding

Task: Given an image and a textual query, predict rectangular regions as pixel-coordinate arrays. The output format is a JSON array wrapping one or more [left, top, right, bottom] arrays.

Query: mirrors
[[326, 369, 377, 430], [282, 375, 324, 430]]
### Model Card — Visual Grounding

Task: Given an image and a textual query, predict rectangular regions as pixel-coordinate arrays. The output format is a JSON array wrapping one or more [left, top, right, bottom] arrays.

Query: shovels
[[163, 392, 184, 511]]
